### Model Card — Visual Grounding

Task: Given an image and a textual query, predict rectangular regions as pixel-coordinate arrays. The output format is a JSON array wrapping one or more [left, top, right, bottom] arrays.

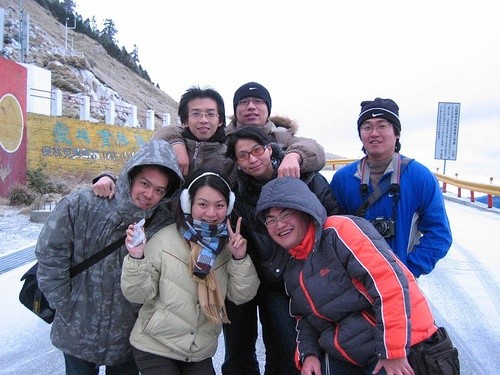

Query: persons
[[18, 73, 463, 375]]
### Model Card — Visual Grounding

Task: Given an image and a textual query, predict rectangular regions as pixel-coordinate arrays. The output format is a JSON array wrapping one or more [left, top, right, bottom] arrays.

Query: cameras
[[367, 216, 395, 237]]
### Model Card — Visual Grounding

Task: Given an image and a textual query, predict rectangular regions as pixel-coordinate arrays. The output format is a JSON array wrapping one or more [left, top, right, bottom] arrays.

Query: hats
[[357, 97, 401, 140], [233, 82, 271, 118]]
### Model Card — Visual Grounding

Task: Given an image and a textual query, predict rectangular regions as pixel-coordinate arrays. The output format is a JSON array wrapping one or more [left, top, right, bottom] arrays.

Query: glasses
[[187, 112, 219, 118], [236, 144, 270, 162], [238, 98, 266, 105], [263, 210, 298, 228]]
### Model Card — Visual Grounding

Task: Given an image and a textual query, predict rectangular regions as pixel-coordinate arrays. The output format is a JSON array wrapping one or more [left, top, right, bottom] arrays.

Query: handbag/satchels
[[18, 262, 56, 324]]
